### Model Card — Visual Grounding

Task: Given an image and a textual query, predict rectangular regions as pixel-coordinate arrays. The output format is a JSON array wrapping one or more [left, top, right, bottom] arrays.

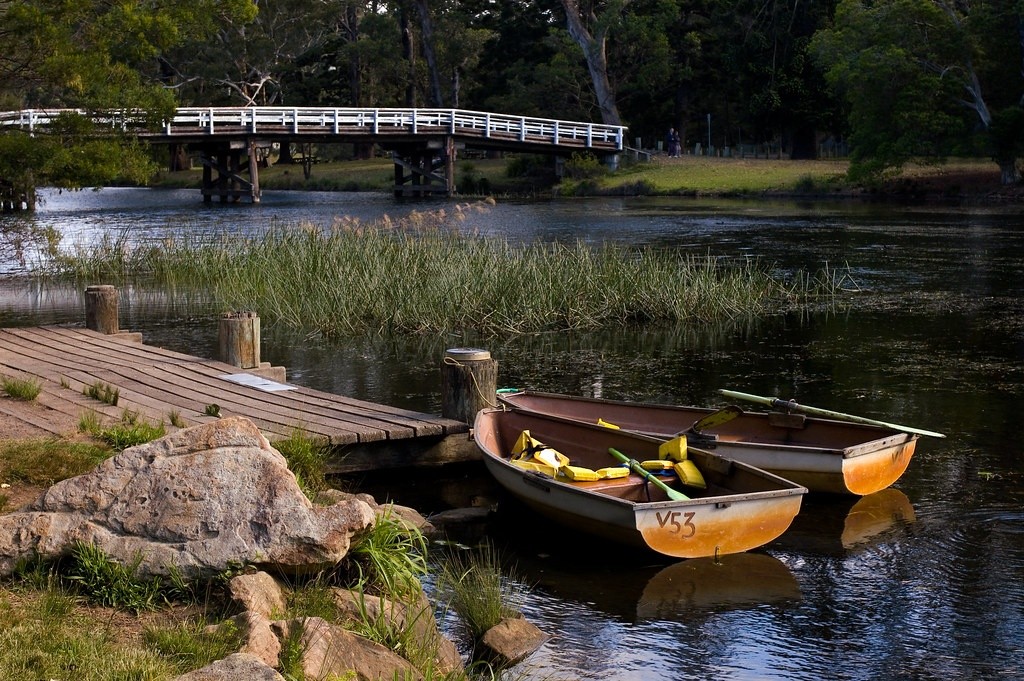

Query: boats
[[472, 407, 809, 561], [752, 486, 915, 558], [497, 387, 920, 496], [497, 489, 801, 623]]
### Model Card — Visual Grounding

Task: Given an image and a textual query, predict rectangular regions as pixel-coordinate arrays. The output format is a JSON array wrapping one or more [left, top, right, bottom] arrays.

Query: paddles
[[609, 446, 691, 501], [674, 404, 744, 437], [718, 388, 947, 439]]
